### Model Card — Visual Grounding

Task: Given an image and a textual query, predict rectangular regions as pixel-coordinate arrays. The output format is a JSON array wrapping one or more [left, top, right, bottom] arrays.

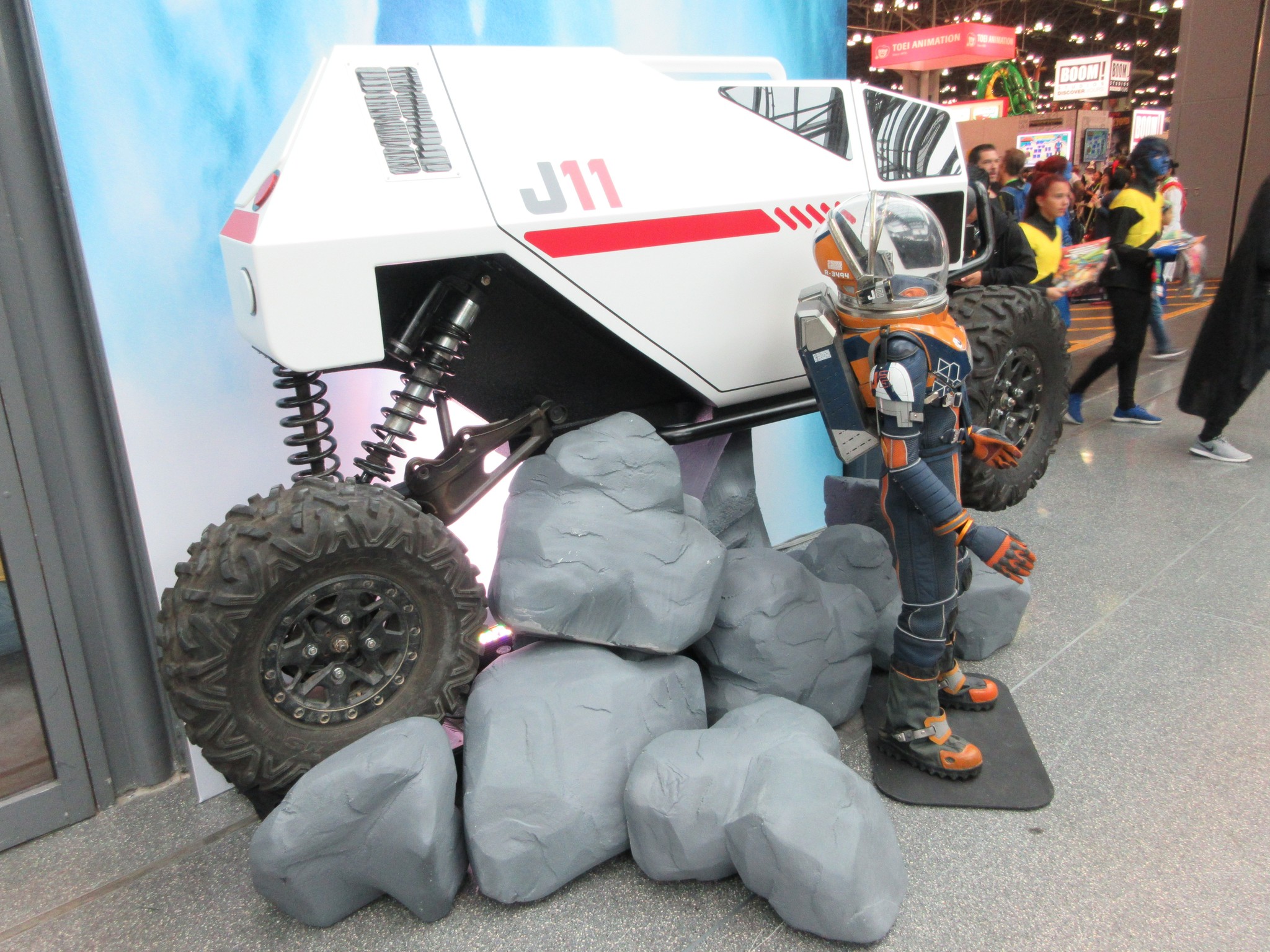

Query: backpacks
[[999, 182, 1031, 222]]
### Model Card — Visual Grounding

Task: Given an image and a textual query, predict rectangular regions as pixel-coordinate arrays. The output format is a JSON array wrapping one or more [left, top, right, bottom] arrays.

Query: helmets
[[814, 189, 948, 330], [1130, 136, 1170, 161]]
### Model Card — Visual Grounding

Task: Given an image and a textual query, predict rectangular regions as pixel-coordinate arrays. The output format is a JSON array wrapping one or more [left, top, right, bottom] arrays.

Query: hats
[[1087, 161, 1096, 169]]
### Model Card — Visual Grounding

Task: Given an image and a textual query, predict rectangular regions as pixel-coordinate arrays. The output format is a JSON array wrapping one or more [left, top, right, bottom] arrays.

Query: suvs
[[154, 41, 1075, 796]]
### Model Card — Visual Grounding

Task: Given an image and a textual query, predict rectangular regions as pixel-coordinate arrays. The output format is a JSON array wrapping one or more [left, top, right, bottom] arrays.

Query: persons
[[1176, 174, 1270, 463], [954, 134, 1207, 360], [792, 187, 1040, 782], [1060, 136, 1181, 427]]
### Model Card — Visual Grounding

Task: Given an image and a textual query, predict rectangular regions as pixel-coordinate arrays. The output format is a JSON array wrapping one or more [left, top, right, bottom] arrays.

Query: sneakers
[[1064, 392, 1083, 427], [1111, 404, 1162, 424], [1189, 431, 1252, 462]]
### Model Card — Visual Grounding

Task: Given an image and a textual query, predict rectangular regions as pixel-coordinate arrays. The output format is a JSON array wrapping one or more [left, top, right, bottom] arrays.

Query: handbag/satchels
[[1069, 205, 1085, 245]]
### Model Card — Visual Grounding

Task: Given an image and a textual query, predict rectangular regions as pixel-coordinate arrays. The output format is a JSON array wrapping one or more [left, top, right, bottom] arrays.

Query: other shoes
[[1149, 346, 1188, 360]]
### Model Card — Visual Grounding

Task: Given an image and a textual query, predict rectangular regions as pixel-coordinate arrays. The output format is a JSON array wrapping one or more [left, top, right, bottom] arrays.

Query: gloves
[[958, 524, 1036, 584], [966, 424, 1023, 471], [1151, 245, 1180, 262]]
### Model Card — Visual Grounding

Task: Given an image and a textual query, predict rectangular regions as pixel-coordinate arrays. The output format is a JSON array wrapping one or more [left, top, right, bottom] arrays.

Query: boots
[[877, 665, 984, 780], [938, 632, 999, 713]]
